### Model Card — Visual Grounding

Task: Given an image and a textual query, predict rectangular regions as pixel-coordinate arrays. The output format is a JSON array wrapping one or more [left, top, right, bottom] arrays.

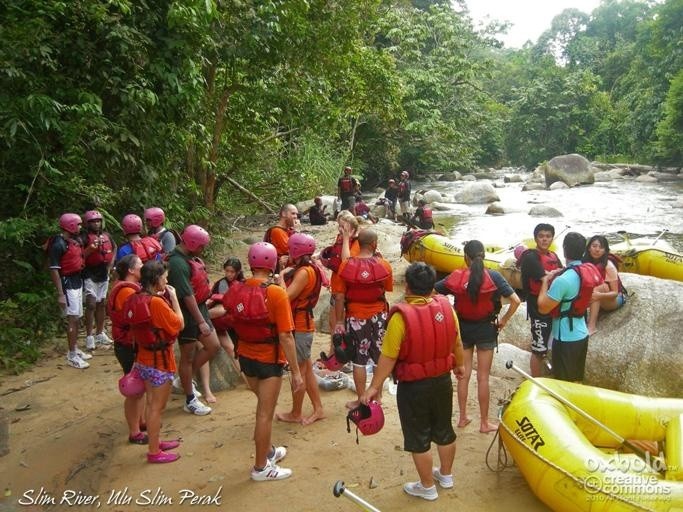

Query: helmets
[[248, 242, 277, 274], [122, 214, 143, 236], [320, 246, 337, 267], [315, 197, 321, 205], [418, 196, 426, 205], [515, 245, 528, 259], [82, 210, 102, 228], [320, 342, 347, 371], [349, 401, 384, 435], [287, 233, 315, 259], [143, 207, 165, 228], [181, 225, 210, 252], [388, 179, 395, 184], [344, 166, 351, 174], [119, 361, 145, 397], [60, 213, 82, 235]]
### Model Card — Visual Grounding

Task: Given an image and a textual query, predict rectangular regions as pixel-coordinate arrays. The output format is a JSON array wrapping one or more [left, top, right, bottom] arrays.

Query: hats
[[401, 171, 409, 178]]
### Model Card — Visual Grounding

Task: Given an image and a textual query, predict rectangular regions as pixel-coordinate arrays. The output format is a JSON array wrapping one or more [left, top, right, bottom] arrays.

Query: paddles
[[505, 359, 666, 477]]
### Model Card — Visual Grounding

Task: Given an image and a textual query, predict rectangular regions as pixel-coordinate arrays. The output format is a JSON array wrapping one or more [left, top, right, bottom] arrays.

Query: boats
[[609, 237, 683, 283], [497, 377, 683, 512], [400, 229, 558, 290]]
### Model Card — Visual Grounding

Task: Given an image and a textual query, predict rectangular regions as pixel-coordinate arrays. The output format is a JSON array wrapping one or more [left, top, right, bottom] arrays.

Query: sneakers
[[140, 420, 163, 431], [68, 356, 90, 369], [173, 375, 202, 397], [183, 396, 212, 416], [432, 467, 453, 488], [251, 457, 292, 481], [147, 449, 180, 463], [404, 480, 439, 500], [268, 444, 286, 464], [159, 441, 179, 451], [128, 432, 150, 444], [85, 336, 96, 350], [66, 347, 92, 360], [95, 332, 113, 344]]
